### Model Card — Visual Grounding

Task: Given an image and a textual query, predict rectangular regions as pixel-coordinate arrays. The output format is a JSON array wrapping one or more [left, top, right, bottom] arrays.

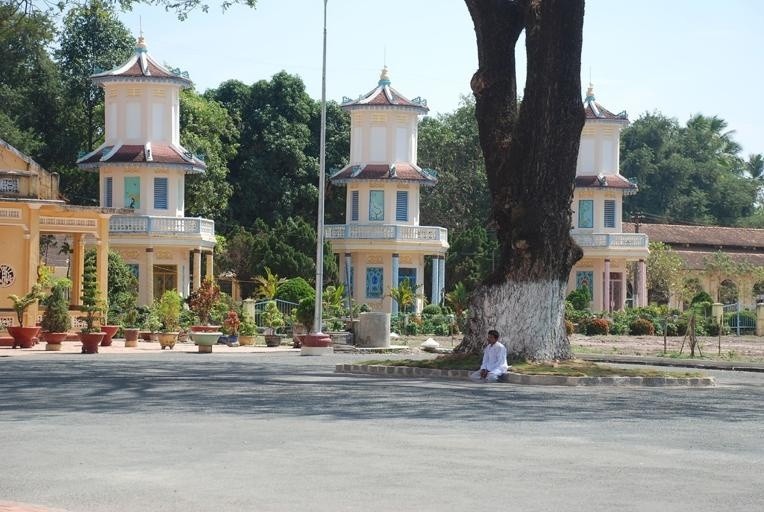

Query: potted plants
[[224, 309, 241, 342], [291, 300, 315, 347], [261, 302, 285, 347], [239, 302, 258, 345], [157, 288, 182, 349], [39, 276, 73, 344], [7, 260, 49, 349], [122, 308, 141, 347], [179, 295, 192, 342], [185, 275, 224, 345], [147, 297, 163, 342], [99, 298, 122, 346], [75, 255, 107, 352]]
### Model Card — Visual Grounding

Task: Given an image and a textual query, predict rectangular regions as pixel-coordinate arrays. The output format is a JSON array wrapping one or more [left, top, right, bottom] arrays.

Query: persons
[[469, 330, 508, 383]]
